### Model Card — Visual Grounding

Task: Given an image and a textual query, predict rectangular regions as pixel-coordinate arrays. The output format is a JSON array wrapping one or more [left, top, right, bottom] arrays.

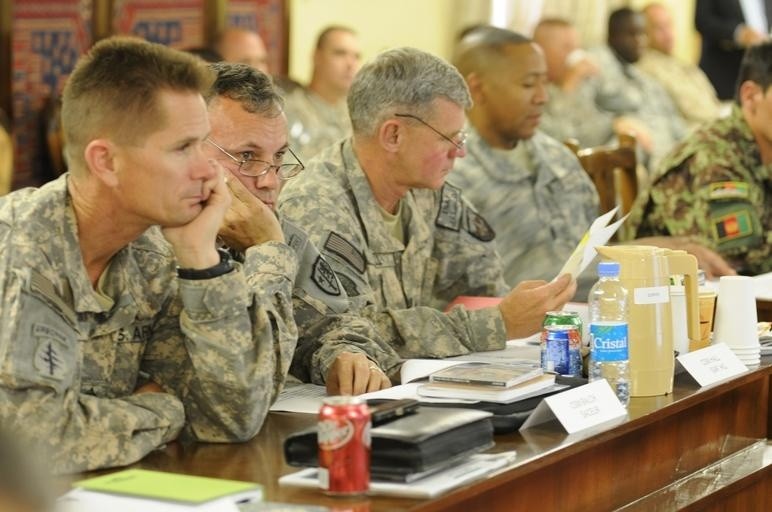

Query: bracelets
[[168, 248, 235, 281]]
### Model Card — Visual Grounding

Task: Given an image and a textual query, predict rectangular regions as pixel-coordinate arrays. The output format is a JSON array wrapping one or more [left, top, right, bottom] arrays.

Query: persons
[[278, 44, 580, 361], [693, 0, 772, 105], [529, 10, 660, 155], [206, 25, 339, 156], [586, 3, 688, 176], [613, 36, 772, 276], [642, 2, 722, 129], [178, 44, 224, 68], [0, 36, 280, 511], [306, 25, 363, 141], [198, 56, 395, 400], [446, 23, 620, 302]]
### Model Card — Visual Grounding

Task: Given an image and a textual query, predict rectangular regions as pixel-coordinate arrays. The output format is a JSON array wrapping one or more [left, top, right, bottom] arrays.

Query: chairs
[[564, 134, 639, 235]]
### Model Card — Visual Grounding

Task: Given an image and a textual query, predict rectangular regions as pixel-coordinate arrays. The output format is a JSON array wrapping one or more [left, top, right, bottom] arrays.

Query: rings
[[368, 366, 385, 374]]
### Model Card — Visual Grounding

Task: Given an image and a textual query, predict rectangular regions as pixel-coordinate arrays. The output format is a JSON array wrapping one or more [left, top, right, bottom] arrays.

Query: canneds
[[542, 311, 582, 336], [541, 325, 583, 378], [318, 396, 371, 496]]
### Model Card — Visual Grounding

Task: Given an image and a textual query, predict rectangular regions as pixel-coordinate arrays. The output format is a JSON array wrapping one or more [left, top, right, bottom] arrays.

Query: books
[[58, 464, 270, 512], [274, 448, 520, 503], [416, 374, 559, 406], [428, 362, 546, 389], [281, 404, 498, 486]]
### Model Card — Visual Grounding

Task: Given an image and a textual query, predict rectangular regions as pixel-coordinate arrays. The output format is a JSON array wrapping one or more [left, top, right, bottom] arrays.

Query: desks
[[48, 310, 772, 512]]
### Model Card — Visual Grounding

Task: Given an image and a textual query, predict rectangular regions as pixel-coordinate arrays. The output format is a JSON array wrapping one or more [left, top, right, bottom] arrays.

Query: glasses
[[395, 113, 467, 147], [206, 138, 305, 180]]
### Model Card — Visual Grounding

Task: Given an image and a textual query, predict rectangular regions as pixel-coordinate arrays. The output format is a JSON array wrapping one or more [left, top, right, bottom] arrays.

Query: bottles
[[587, 264, 631, 411]]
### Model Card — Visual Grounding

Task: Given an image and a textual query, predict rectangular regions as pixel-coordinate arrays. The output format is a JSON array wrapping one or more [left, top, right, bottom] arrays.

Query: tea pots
[[592, 244, 702, 398]]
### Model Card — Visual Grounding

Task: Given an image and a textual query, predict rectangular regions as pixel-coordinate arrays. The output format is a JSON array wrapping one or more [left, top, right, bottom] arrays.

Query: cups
[[673, 286, 715, 349], [715, 273, 760, 370]]
